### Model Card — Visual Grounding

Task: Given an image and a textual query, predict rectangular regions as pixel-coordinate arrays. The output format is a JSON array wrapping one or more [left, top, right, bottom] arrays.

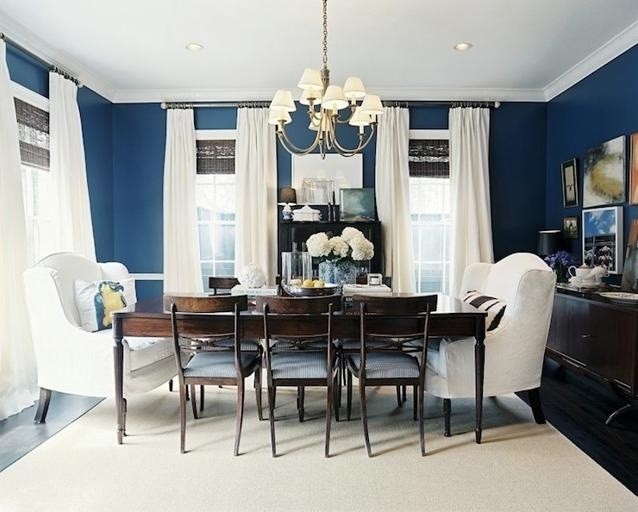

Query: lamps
[[536, 230, 564, 256], [265, 0, 392, 158]]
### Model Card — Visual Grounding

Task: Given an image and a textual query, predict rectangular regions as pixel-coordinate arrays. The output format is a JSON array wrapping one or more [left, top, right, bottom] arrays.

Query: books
[[343, 284, 392, 297], [282, 251, 318, 280], [231, 284, 280, 300]]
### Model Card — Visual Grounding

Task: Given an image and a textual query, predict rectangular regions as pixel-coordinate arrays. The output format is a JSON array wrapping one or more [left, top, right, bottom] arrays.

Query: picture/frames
[[562, 217, 578, 240], [559, 158, 579, 209], [629, 132, 638, 207], [581, 134, 627, 209], [580, 206, 623, 275]]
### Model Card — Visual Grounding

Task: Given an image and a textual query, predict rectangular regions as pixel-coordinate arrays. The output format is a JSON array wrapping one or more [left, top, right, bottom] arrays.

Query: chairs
[[160, 292, 263, 457], [252, 294, 341, 459], [355, 275, 392, 288], [207, 274, 240, 296], [346, 294, 438, 457], [406, 248, 558, 428], [21, 253, 194, 423]]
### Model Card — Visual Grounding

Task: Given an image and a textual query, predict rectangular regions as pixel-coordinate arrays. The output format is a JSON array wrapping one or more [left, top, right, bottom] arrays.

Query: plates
[[595, 292, 638, 304], [283, 284, 339, 297]]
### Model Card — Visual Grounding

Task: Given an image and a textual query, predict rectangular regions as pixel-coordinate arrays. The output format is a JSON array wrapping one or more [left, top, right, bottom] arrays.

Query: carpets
[[1, 380, 638, 511]]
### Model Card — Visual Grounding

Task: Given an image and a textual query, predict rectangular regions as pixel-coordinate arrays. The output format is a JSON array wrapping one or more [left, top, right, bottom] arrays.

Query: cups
[[328, 204, 340, 223]]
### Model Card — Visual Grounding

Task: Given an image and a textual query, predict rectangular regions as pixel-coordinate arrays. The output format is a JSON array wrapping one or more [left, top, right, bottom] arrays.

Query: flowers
[[305, 226, 374, 264], [543, 250, 578, 269]]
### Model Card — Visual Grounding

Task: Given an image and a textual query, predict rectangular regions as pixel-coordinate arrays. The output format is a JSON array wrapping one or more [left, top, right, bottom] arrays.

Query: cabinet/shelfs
[[543, 290, 638, 427]]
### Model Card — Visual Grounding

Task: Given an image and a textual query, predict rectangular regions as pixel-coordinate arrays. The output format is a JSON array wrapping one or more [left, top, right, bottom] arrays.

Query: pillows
[[463, 287, 506, 331], [76, 277, 128, 333]]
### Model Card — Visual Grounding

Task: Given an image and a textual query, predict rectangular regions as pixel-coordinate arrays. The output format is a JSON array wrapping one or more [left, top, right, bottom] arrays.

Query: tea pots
[[568, 262, 591, 279]]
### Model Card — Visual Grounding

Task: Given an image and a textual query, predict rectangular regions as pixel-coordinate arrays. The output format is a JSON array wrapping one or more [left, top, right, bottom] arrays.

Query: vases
[[317, 257, 356, 291], [557, 263, 568, 293]]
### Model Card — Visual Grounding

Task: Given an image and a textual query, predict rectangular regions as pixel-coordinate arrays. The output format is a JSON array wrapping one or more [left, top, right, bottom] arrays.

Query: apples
[[303, 279, 325, 289]]
